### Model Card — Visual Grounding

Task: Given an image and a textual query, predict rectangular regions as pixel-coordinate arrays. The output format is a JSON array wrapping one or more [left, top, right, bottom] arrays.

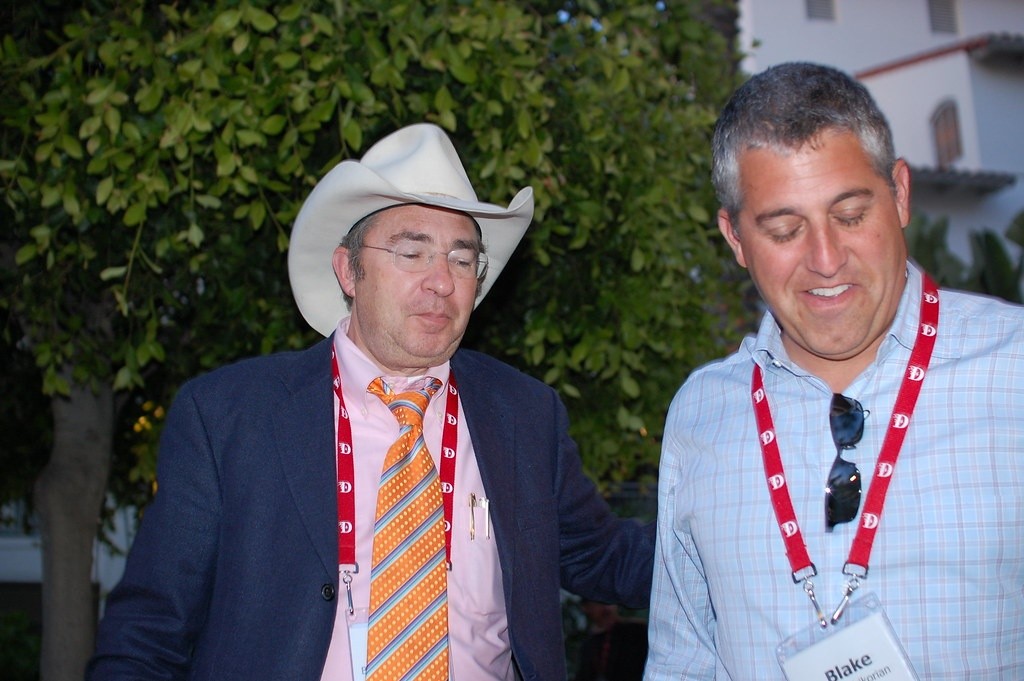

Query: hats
[[287, 123, 535, 338]]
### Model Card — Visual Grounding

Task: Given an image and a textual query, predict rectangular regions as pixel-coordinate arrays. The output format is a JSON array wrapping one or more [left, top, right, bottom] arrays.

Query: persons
[[80, 122, 656, 681], [646, 63, 1024, 681]]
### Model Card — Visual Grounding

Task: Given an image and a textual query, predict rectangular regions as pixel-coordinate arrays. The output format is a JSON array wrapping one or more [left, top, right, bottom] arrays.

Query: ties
[[366, 376, 451, 681]]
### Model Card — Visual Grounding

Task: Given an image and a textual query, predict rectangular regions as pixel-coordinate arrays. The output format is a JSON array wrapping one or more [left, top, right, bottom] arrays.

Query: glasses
[[825, 392, 872, 532], [361, 242, 489, 279]]
